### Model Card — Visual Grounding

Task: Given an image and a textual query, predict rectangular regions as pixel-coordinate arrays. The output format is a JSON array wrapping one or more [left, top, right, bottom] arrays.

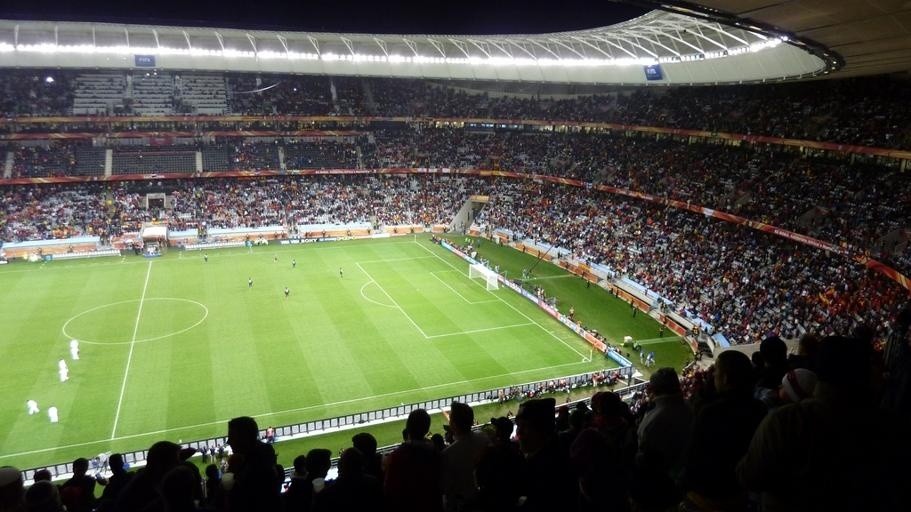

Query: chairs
[[7, 145, 353, 177]]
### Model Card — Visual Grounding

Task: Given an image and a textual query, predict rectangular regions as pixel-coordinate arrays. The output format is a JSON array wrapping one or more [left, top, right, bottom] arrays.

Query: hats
[[780, 368, 818, 403]]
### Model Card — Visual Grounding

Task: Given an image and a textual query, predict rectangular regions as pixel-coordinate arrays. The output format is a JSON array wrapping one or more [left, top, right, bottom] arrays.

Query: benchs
[[70, 68, 229, 119]]
